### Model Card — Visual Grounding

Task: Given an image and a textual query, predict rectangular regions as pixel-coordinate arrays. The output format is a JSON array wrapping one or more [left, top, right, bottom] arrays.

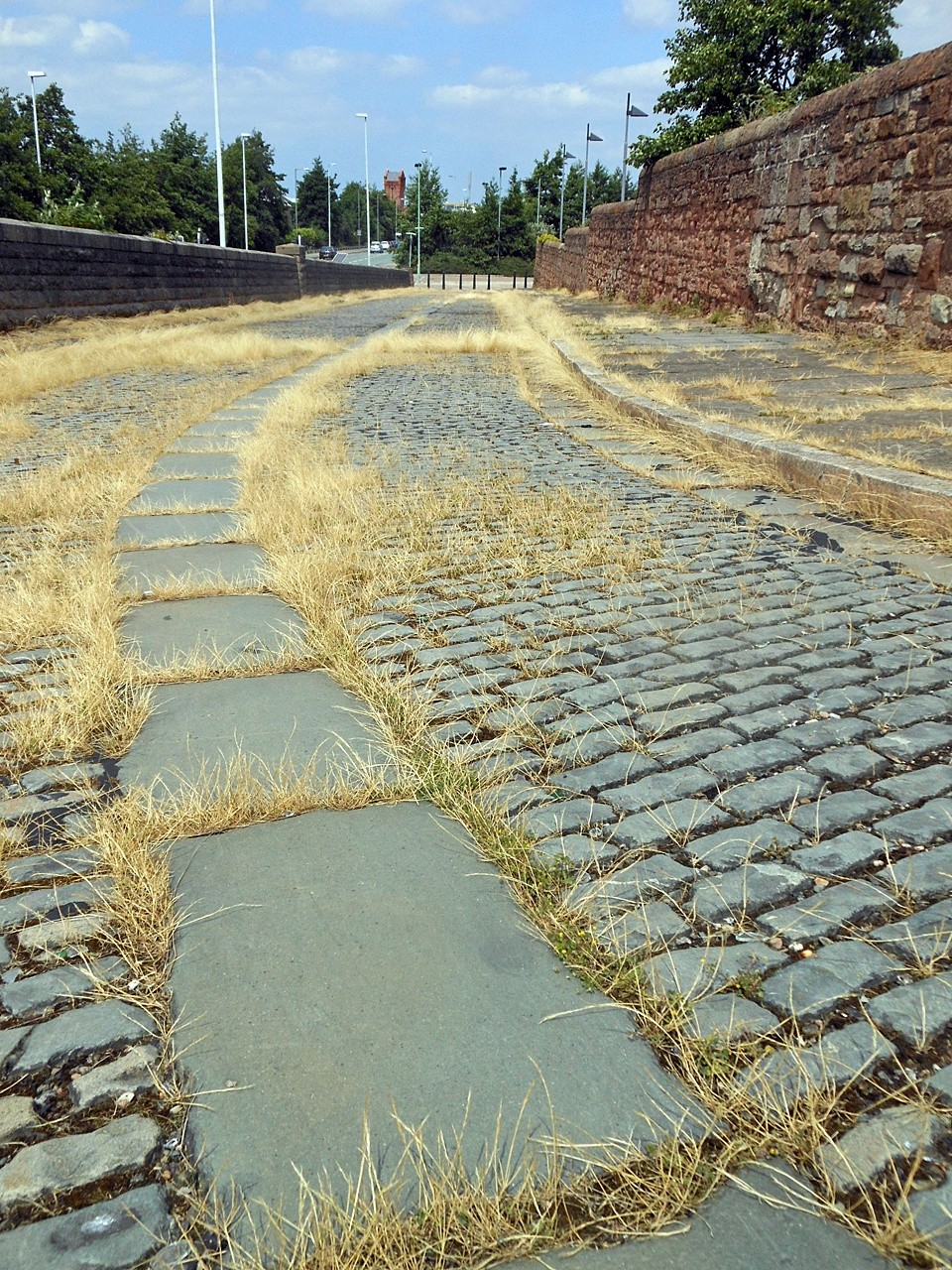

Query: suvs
[[319, 246, 337, 259]]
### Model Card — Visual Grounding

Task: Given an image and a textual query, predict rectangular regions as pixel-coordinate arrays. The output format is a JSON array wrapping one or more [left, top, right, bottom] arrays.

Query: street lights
[[420, 149, 433, 181], [354, 112, 372, 267], [405, 231, 416, 267], [28, 69, 48, 207], [327, 163, 337, 248], [414, 227, 424, 283], [240, 133, 251, 251], [294, 167, 310, 242]]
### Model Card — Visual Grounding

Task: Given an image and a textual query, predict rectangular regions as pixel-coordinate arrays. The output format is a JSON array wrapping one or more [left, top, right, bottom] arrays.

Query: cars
[[389, 241, 399, 248]]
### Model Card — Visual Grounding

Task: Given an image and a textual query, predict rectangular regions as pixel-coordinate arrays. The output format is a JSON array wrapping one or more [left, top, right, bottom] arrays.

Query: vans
[[370, 241, 384, 254], [381, 241, 389, 249]]
